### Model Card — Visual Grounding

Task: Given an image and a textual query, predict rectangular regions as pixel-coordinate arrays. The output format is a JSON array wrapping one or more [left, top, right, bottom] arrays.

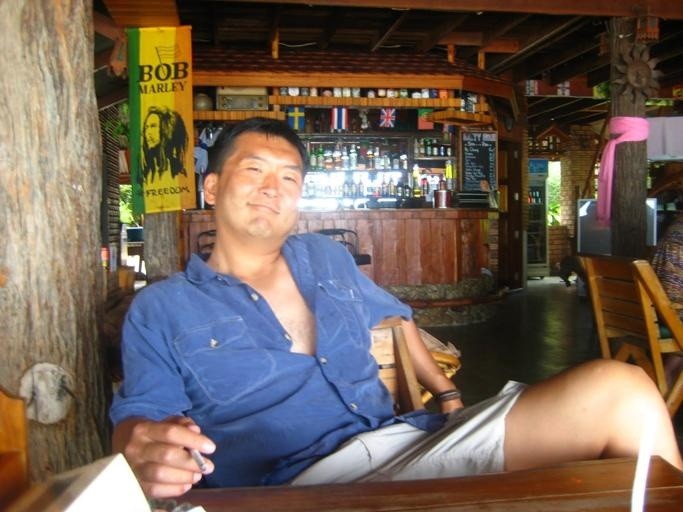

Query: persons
[[648, 208, 682, 392], [138, 107, 187, 179], [106, 114, 681, 503]]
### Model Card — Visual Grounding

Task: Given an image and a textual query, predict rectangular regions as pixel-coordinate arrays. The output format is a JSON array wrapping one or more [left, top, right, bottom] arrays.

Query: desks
[[155, 454, 682, 512]]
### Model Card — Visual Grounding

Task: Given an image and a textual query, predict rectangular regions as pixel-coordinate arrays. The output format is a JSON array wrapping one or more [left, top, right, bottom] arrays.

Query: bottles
[[301, 138, 454, 200], [434, 179, 452, 208], [193, 93, 213, 111], [526, 134, 561, 153], [279, 86, 448, 99]]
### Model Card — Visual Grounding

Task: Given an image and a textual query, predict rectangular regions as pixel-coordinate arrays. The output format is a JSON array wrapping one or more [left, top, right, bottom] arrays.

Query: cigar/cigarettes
[[185, 446, 207, 471]]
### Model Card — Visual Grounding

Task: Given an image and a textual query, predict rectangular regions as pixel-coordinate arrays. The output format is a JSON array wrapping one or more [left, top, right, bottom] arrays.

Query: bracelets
[[435, 386, 463, 403]]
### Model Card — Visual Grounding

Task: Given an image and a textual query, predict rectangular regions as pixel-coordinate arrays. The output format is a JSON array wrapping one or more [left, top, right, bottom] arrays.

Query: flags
[[380, 108, 395, 129], [331, 105, 350, 130], [285, 105, 305, 130], [417, 108, 433, 130]]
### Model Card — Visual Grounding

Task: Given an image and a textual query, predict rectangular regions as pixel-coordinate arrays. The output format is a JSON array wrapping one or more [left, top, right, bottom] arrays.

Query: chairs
[[575, 253, 683, 415]]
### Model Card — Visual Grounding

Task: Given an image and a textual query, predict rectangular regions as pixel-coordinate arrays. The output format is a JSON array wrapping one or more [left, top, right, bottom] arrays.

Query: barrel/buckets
[[117, 263, 136, 290], [433, 189, 453, 209]]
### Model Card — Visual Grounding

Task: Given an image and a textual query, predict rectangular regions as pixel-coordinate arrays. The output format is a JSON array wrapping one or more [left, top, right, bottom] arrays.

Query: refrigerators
[[526, 158, 548, 278]]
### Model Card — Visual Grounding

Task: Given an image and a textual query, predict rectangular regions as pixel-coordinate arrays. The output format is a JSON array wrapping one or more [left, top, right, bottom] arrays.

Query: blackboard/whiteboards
[[460, 131, 498, 191]]
